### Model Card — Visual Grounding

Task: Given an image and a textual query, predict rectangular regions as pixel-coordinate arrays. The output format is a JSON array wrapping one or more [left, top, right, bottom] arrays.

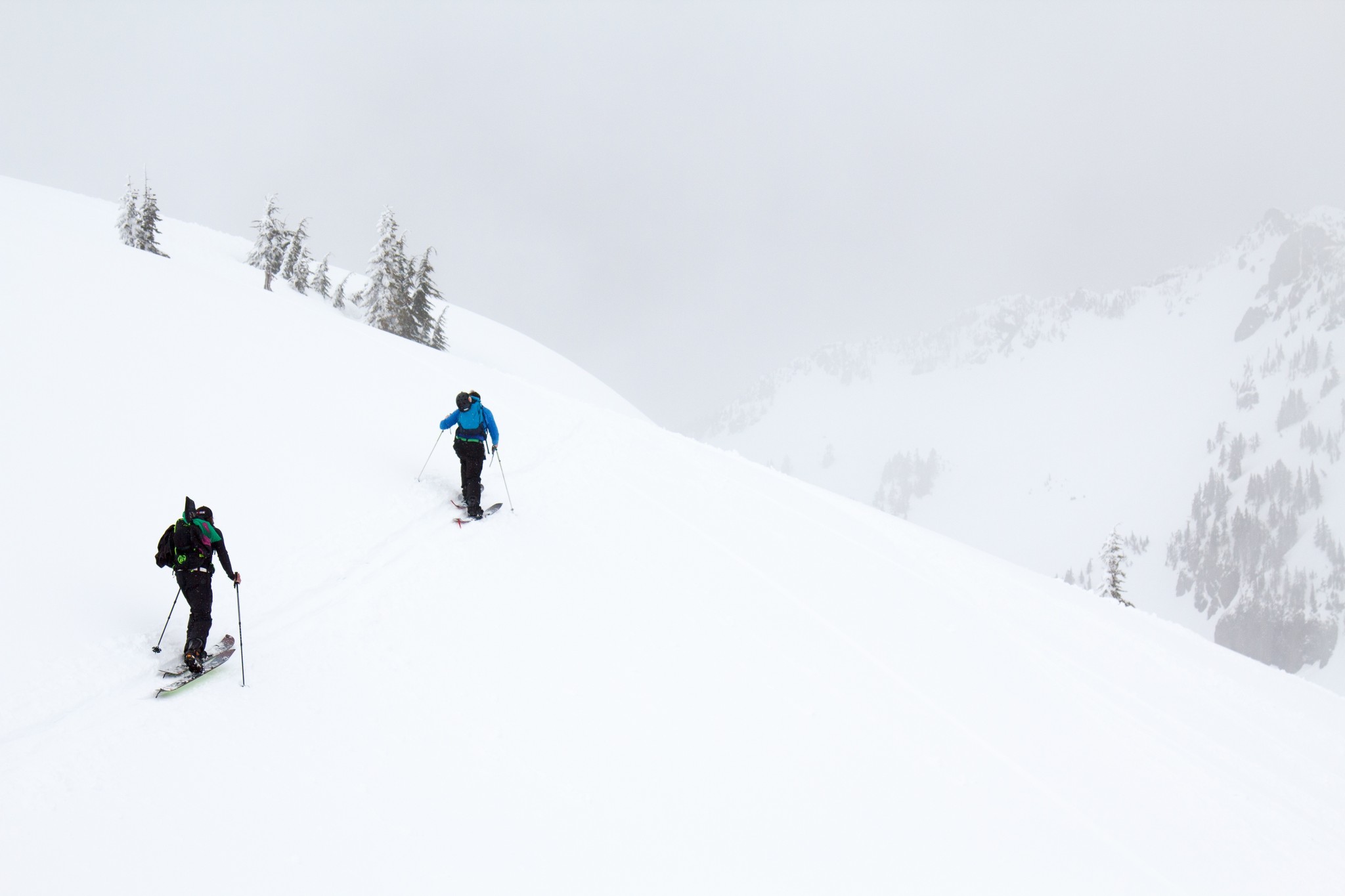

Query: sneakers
[[186, 649, 207, 660], [185, 652, 204, 675]]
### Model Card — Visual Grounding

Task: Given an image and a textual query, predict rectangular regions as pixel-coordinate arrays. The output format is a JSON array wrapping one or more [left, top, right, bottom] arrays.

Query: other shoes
[[459, 500, 468, 507], [468, 509, 484, 517]]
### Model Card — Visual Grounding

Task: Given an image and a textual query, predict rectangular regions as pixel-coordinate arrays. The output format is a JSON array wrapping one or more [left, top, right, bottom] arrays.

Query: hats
[[196, 506, 213, 518]]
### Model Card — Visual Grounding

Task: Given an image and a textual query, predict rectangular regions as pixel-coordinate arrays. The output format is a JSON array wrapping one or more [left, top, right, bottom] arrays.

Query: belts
[[456, 437, 480, 442], [177, 567, 210, 573]]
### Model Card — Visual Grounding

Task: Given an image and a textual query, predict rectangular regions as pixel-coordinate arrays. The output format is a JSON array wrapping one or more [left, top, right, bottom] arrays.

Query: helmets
[[456, 391, 472, 412]]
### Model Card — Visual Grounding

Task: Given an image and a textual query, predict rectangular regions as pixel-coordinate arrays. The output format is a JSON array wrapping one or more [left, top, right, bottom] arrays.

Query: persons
[[440, 392, 499, 518], [155, 506, 241, 674]]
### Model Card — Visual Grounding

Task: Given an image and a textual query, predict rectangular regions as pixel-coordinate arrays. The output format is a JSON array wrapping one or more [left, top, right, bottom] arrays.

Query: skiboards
[[157, 634, 236, 692], [453, 484, 503, 523]]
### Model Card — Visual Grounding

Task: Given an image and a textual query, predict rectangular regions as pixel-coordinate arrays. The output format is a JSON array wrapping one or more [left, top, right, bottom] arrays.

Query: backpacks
[[172, 518, 215, 576], [458, 402, 488, 437]]
